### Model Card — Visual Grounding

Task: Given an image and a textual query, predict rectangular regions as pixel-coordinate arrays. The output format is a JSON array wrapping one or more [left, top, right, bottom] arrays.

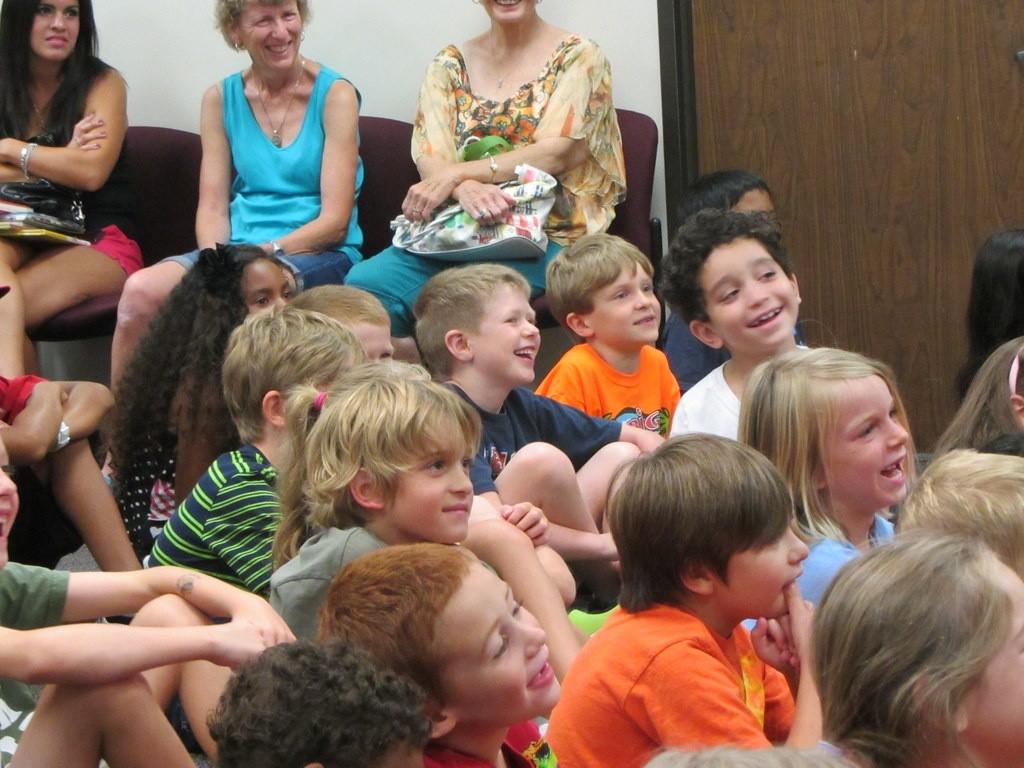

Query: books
[[0, 202, 93, 247]]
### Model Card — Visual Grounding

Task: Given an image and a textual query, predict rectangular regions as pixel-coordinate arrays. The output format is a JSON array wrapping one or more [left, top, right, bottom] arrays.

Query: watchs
[[271, 239, 285, 259]]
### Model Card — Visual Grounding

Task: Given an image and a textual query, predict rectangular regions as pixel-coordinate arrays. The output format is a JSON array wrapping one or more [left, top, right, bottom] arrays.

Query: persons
[[102, 0, 365, 471], [342, 1, 627, 364], [0, 0, 150, 377], [0, 169, 1024, 768]]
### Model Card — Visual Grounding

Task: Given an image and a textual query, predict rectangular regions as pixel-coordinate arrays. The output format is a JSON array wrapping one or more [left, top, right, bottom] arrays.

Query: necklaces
[[489, 30, 535, 88], [252, 60, 305, 146]]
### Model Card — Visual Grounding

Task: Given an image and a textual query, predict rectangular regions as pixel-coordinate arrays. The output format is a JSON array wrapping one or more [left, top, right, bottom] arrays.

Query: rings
[[480, 209, 488, 217], [75, 137, 82, 145]]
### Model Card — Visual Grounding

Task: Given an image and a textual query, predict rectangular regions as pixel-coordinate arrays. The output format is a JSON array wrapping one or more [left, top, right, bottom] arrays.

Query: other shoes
[[96, 615, 135, 624]]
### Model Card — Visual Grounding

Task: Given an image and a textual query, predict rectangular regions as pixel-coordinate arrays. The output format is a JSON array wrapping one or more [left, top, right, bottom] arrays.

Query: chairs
[[32, 110, 663, 338]]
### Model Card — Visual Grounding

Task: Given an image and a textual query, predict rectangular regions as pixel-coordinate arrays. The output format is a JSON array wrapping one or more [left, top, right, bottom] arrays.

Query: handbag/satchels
[[390, 136, 557, 259], [0, 134, 86, 226]]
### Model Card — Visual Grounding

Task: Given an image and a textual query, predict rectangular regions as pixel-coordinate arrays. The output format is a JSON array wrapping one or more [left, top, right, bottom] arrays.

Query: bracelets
[[19, 143, 37, 179], [487, 156, 497, 183], [50, 421, 71, 453]]
